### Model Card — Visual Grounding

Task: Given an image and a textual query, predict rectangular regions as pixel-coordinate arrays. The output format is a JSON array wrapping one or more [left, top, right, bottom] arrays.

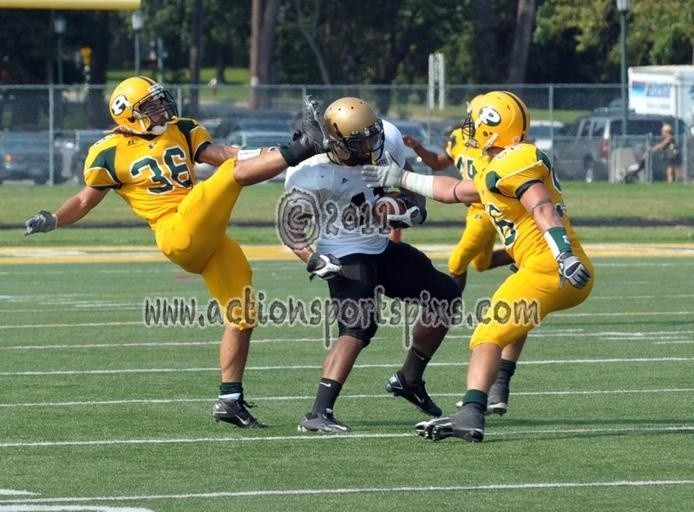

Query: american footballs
[[373, 192, 408, 229]]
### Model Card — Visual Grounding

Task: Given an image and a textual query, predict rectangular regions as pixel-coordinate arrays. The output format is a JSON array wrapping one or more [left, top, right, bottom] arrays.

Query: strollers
[[617, 153, 655, 183]]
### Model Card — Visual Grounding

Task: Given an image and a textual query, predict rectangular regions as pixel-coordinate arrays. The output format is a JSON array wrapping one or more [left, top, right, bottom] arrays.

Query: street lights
[[613, 0, 635, 134], [52, 13, 71, 128], [128, 7, 146, 75]]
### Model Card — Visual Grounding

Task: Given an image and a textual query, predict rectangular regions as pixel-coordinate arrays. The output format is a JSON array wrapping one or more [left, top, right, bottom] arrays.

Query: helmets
[[109, 75, 178, 135], [323, 97, 385, 166], [461, 90, 532, 149]]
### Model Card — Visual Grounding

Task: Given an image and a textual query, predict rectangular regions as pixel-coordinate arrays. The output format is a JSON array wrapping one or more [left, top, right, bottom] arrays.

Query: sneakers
[[296, 408, 350, 433], [295, 94, 330, 154], [415, 406, 486, 442], [455, 378, 509, 416], [212, 392, 268, 429], [385, 371, 442, 417]]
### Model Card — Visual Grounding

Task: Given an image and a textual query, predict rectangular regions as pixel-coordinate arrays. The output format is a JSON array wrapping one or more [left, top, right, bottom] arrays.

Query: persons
[[414, 90, 594, 443], [285, 97, 462, 435], [400, 94, 515, 307], [24, 77, 332, 429], [650, 123, 694, 185]]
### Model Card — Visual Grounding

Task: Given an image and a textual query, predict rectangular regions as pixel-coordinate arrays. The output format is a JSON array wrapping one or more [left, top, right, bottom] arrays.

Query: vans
[[545, 107, 694, 181]]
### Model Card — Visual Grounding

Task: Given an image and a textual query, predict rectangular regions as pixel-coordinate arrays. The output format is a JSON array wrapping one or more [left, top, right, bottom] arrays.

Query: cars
[[1, 106, 563, 192]]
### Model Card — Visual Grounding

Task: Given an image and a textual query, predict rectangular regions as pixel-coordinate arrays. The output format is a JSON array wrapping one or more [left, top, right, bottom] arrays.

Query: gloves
[[361, 150, 404, 188], [24, 210, 56, 236], [555, 251, 591, 289]]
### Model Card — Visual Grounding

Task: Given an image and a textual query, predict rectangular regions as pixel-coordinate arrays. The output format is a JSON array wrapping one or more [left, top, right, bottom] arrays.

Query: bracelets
[[236, 148, 262, 160], [544, 226, 572, 259], [403, 171, 433, 200]]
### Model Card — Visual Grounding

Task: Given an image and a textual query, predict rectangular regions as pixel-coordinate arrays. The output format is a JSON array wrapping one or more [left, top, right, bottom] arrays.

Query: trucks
[[628, 64, 694, 137]]
